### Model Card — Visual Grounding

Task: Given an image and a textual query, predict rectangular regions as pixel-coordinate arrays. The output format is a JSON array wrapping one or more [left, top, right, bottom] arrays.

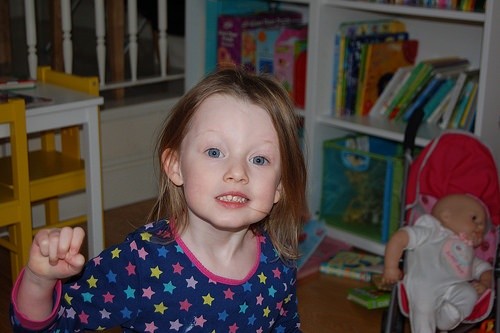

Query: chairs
[[0, 64, 104, 286]]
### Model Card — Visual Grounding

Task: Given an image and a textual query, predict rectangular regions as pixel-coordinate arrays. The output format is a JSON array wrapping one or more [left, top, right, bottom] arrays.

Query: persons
[[10, 68, 306, 333], [382, 194, 495, 333]]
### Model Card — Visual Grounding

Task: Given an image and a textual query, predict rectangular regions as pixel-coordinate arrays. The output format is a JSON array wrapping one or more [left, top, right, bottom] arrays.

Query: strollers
[[381, 108, 500, 333]]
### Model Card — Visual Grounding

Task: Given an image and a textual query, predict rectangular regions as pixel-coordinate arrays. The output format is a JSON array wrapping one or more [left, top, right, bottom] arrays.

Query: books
[[294, 1, 486, 309]]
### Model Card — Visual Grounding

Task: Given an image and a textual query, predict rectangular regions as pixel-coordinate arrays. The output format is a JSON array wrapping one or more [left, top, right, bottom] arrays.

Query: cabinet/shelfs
[[185, 0, 499, 256]]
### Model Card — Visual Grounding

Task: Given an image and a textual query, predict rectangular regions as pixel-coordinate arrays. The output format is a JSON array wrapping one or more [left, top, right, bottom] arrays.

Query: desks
[[0, 79, 104, 265]]
